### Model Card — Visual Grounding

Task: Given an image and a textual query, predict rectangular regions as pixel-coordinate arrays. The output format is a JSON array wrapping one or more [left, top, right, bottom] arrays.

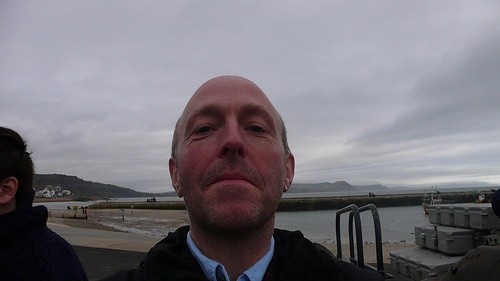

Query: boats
[[422, 186, 442, 214]]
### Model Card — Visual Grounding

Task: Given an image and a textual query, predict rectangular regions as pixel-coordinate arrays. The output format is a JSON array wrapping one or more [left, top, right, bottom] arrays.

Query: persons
[[0, 126, 88, 281], [369, 191, 375, 197], [491, 188, 500, 219], [120, 210, 124, 222], [96, 75, 385, 281]]
